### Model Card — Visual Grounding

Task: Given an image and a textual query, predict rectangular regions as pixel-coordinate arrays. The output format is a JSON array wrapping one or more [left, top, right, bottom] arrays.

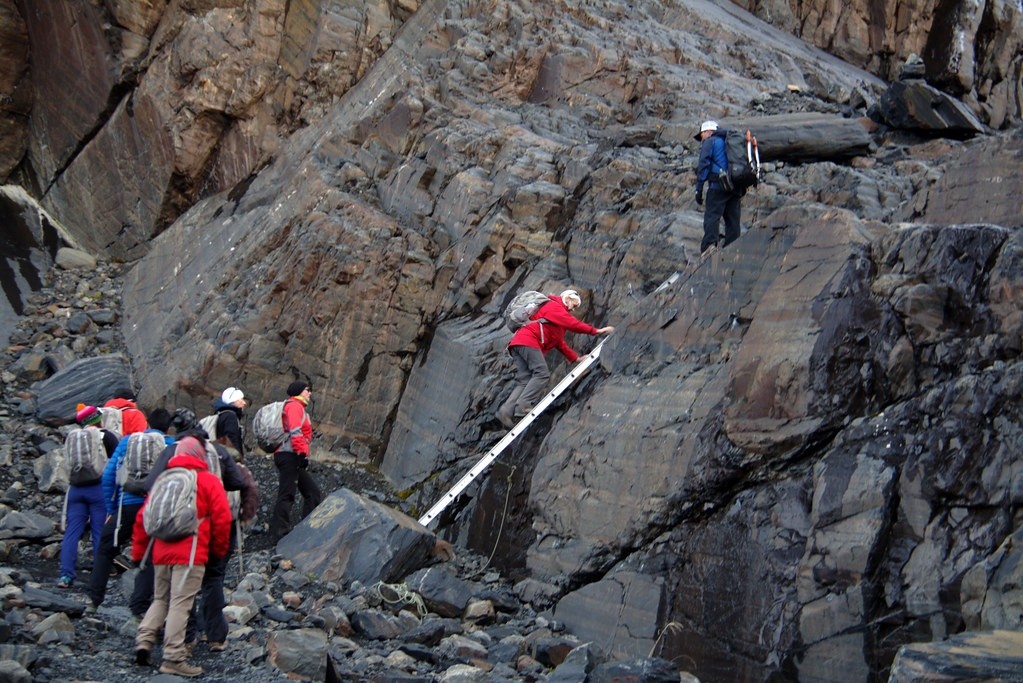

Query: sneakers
[[137, 641, 154, 665], [158, 659, 202, 677], [113, 555, 135, 571], [57, 575, 71, 588], [199, 630, 228, 652], [182, 636, 198, 656]]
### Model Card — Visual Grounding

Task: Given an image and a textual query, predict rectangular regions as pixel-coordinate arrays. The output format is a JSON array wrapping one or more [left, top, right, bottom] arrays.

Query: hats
[[693, 121, 720, 141], [76, 381, 311, 458]]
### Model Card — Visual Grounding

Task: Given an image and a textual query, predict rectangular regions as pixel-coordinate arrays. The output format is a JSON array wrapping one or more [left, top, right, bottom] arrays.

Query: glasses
[[569, 293, 578, 296]]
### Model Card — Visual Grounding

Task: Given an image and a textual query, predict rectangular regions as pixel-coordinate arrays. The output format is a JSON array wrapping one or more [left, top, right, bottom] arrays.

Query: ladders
[[416, 270, 681, 528]]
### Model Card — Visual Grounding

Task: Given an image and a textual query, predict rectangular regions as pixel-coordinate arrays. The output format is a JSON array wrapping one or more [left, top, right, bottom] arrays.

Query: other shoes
[[701, 244, 716, 257], [515, 405, 543, 417], [85, 552, 118, 577], [496, 408, 515, 429], [135, 612, 167, 627]]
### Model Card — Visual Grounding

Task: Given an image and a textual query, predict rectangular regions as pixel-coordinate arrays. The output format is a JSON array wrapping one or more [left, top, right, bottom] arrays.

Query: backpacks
[[143, 465, 207, 542], [98, 406, 134, 436], [64, 424, 110, 487], [173, 438, 223, 487], [700, 129, 760, 187], [197, 409, 239, 443], [115, 431, 171, 494], [218, 460, 244, 522], [504, 290, 557, 333], [253, 400, 306, 452]]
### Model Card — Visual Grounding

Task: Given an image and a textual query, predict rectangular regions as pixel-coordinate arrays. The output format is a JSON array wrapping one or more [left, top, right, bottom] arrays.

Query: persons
[[57, 403, 118, 589], [131, 436, 230, 677], [494, 289, 614, 430], [86, 385, 260, 650], [271, 381, 320, 544], [693, 120, 740, 251]]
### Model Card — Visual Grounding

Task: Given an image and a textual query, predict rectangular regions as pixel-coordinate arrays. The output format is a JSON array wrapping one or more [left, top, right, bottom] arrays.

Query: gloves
[[296, 453, 308, 469], [696, 190, 703, 205]]
